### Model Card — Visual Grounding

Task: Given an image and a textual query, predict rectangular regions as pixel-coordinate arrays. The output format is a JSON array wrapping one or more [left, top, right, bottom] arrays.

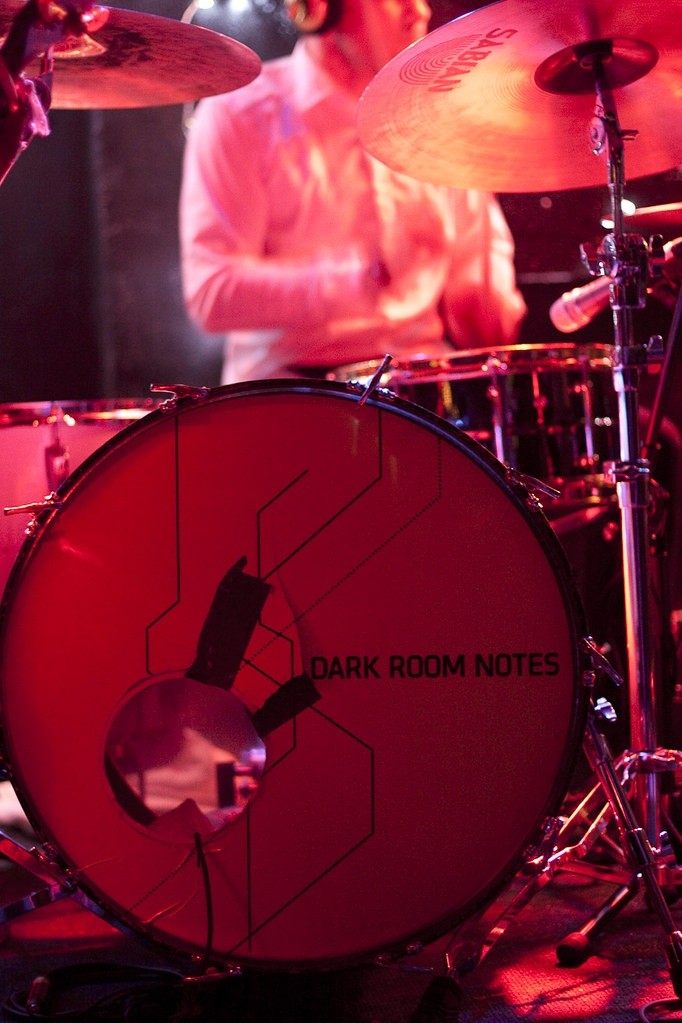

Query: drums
[[325, 342, 622, 510], [0, 395, 171, 596], [0, 377, 594, 980]]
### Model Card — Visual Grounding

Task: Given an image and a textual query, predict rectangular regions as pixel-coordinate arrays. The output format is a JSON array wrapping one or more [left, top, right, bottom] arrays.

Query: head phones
[[283, 0, 338, 35]]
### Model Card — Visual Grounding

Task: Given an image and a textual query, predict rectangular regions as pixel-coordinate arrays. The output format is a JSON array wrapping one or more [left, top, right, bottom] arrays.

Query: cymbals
[[352, 0, 682, 194], [0, 1, 263, 112]]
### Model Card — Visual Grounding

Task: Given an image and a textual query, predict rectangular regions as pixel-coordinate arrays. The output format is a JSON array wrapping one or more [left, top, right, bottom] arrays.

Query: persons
[[176, 0, 527, 391]]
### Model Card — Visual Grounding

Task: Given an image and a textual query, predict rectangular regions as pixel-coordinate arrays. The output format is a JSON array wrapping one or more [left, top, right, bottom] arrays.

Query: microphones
[[550, 238, 682, 333]]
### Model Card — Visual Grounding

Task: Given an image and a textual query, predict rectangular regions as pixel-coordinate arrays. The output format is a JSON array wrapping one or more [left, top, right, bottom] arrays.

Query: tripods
[[413, 64, 682, 1022]]
